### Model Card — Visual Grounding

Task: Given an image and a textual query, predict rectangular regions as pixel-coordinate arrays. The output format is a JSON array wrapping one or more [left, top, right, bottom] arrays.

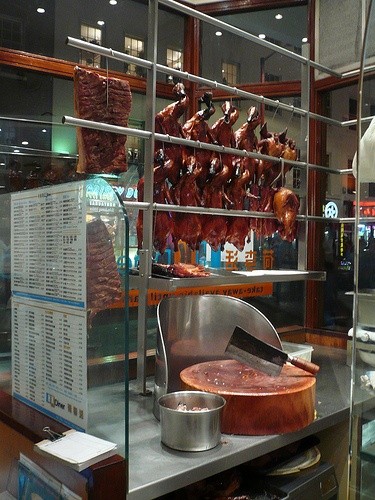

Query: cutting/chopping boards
[[180, 359, 316, 436]]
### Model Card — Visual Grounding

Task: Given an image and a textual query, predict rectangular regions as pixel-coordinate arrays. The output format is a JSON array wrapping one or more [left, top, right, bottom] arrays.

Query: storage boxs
[[281, 342, 314, 363]]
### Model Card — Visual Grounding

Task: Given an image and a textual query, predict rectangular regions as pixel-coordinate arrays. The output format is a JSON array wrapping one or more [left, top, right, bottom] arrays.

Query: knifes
[[223, 325, 320, 379]]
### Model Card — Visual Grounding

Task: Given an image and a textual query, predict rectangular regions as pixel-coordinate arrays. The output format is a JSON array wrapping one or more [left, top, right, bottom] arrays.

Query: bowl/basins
[[156, 390, 227, 452]]
